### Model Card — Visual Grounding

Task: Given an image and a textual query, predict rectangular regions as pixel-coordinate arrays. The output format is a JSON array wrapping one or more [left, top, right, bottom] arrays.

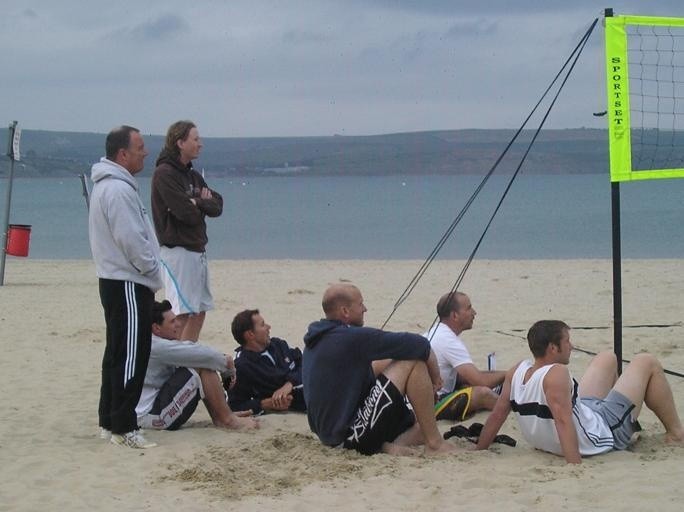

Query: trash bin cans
[[6, 224, 32, 257]]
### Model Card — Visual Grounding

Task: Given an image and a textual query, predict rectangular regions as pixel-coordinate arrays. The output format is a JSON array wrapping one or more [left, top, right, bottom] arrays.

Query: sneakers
[[107, 430, 157, 450], [100, 426, 111, 441]]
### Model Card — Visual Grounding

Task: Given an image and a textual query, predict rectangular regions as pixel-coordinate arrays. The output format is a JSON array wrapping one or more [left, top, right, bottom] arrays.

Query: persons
[[421, 291, 509, 424], [86, 123, 165, 451], [149, 118, 224, 342], [301, 280, 456, 460], [135, 297, 258, 432], [463, 318, 684, 466], [222, 308, 308, 414]]
